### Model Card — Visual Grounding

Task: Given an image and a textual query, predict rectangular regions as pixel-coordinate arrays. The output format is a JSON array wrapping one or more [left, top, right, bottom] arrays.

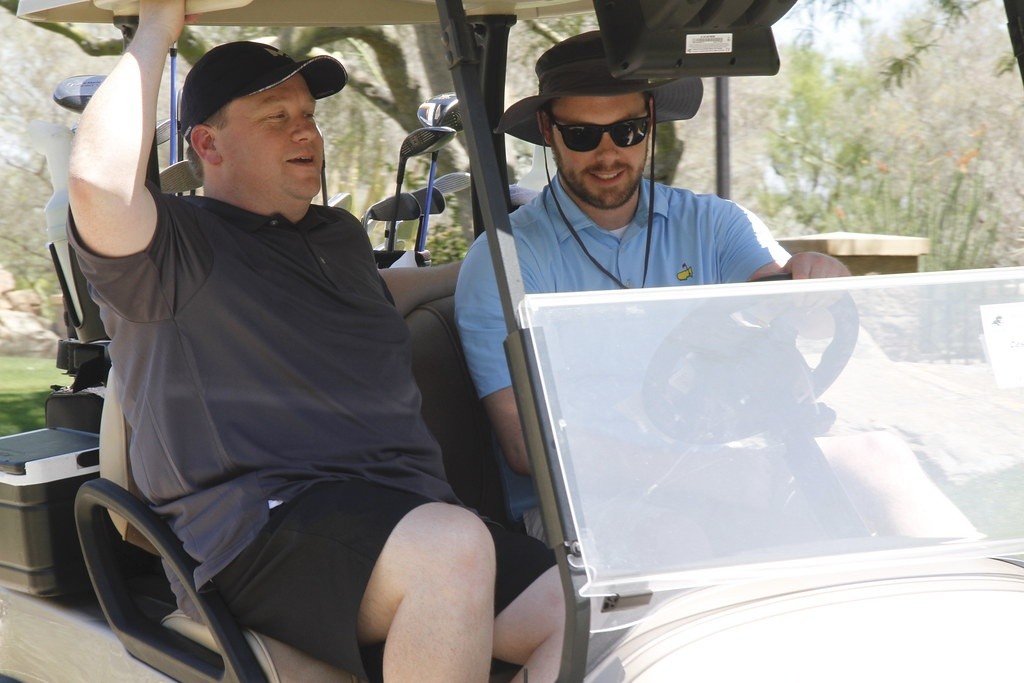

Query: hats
[[179, 40, 348, 141], [493, 30, 704, 147]]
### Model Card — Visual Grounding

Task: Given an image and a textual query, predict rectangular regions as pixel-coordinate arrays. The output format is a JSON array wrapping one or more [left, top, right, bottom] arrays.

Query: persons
[[453, 32, 916, 582], [69, 0, 565, 683]]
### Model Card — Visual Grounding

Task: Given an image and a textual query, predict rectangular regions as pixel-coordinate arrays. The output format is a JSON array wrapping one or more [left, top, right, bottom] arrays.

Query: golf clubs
[[168, 45, 178, 167], [53, 73, 108, 118], [353, 93, 473, 254], [154, 119, 179, 145]]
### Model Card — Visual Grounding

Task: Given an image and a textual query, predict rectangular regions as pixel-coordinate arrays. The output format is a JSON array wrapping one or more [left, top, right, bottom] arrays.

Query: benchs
[[71, 250, 530, 683]]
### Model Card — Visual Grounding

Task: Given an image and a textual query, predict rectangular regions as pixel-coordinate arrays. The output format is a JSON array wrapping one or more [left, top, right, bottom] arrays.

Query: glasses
[[543, 103, 651, 152]]
